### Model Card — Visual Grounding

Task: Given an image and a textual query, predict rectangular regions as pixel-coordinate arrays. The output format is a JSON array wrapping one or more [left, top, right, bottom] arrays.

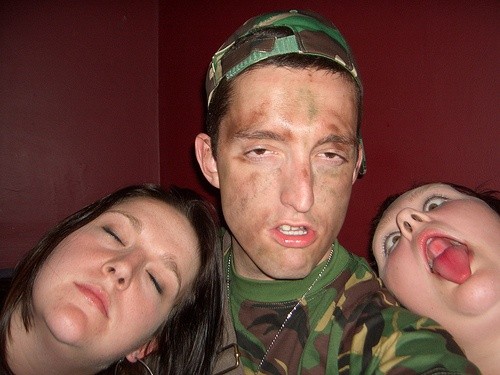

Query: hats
[[206, 10, 367, 175]]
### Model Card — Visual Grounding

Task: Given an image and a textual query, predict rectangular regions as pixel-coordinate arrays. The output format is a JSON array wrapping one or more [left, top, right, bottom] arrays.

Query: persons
[[369, 181, 500, 375], [0, 182, 225, 375], [62, 12, 481, 375]]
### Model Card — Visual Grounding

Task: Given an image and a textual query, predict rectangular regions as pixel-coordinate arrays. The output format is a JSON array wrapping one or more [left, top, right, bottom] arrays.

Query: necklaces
[[225, 239, 334, 375]]
